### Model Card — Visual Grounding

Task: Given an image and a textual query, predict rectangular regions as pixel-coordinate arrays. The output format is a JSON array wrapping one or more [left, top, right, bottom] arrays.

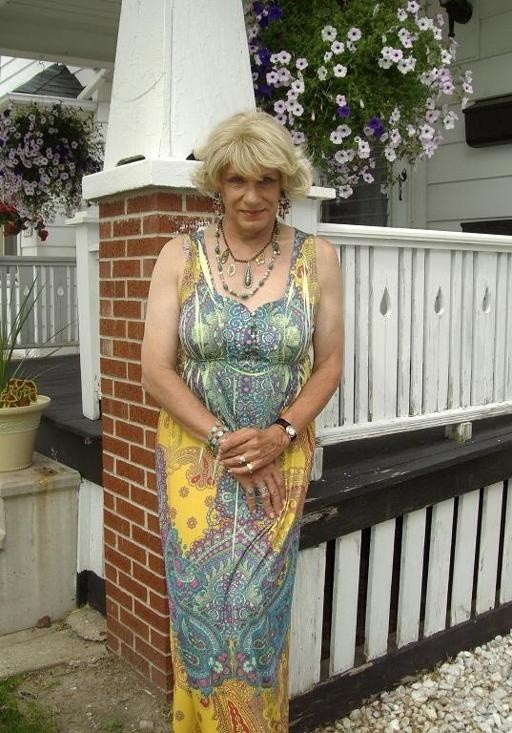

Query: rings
[[246, 462, 254, 474], [237, 455, 247, 467], [245, 488, 257, 497], [257, 485, 269, 497]]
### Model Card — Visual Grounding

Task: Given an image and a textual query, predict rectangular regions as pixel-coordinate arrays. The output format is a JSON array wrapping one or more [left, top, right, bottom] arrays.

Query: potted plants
[[0, 270, 76, 474]]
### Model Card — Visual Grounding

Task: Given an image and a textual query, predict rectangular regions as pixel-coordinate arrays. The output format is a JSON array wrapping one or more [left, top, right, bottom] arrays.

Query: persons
[[140, 109, 346, 733]]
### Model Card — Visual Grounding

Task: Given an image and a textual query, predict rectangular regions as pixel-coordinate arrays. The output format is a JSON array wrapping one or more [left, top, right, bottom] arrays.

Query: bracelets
[[204, 418, 229, 454]]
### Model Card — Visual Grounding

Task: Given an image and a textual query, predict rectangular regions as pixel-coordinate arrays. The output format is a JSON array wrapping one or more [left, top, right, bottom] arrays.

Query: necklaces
[[215, 216, 281, 300]]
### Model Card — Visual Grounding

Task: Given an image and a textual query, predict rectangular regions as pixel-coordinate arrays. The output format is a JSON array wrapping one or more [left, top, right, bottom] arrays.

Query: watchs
[[272, 417, 297, 441]]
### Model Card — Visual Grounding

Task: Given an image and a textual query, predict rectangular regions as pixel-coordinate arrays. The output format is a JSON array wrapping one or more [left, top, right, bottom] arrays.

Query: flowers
[[242, 1, 474, 200], [0, 100, 105, 243]]
